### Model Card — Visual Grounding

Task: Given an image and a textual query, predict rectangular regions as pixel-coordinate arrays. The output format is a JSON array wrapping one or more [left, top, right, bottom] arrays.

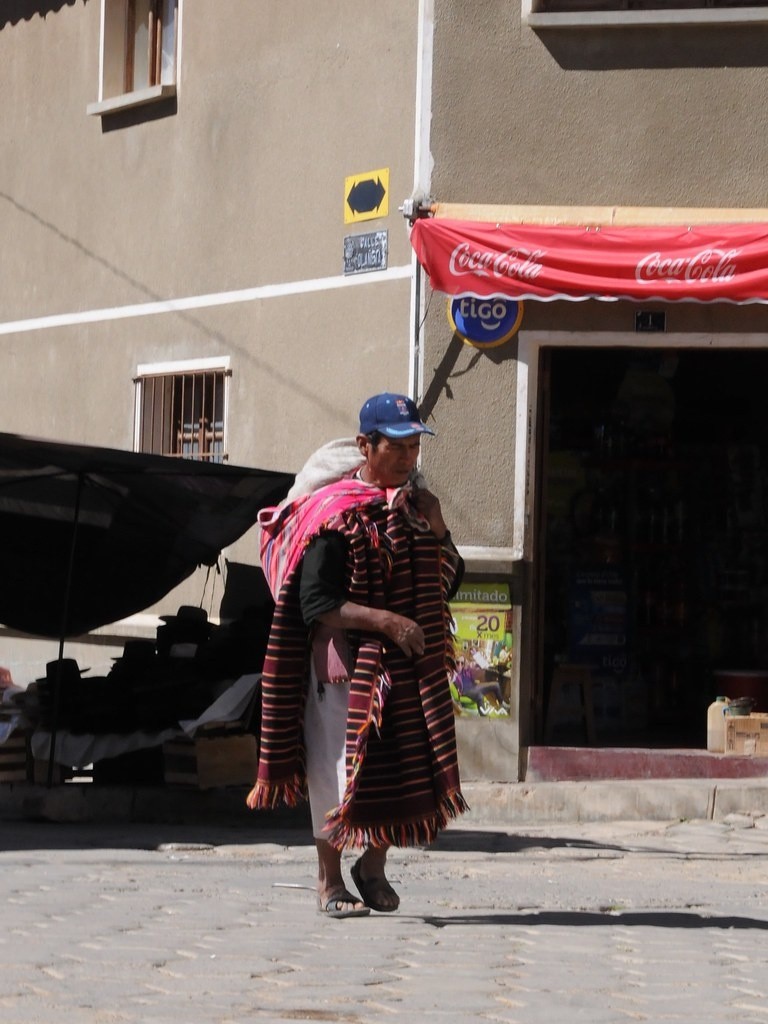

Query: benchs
[[544, 665, 598, 744]]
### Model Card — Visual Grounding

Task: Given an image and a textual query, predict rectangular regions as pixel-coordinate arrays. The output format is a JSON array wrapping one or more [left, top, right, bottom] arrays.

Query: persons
[[260, 392, 471, 918]]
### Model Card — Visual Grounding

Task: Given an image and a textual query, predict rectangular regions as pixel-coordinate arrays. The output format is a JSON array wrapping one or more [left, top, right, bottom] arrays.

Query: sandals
[[350, 857, 400, 912], [316, 888, 371, 918]]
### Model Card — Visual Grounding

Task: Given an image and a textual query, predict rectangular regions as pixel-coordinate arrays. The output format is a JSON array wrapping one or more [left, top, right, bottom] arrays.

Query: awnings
[[408, 213, 768, 305]]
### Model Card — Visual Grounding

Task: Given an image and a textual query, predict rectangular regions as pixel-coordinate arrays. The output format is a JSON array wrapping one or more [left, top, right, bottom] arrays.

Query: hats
[[160, 606, 217, 629], [36, 659, 88, 681], [359, 393, 436, 440], [113, 640, 158, 660]]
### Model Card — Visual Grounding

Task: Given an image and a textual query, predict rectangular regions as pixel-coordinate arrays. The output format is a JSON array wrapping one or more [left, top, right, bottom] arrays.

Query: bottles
[[706, 695, 733, 752]]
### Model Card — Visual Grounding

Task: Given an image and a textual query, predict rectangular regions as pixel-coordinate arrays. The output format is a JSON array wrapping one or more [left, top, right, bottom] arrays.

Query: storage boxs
[[724, 713, 768, 757]]
